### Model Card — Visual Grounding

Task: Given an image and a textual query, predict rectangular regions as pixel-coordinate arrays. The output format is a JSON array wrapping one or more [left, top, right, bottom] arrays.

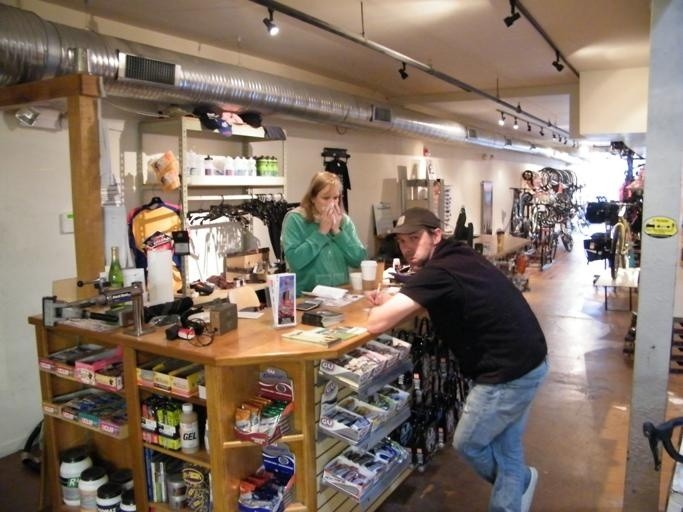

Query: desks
[[594, 267, 640, 311]]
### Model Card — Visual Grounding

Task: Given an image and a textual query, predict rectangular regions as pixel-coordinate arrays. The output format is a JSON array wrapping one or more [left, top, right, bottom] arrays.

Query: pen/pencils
[[377, 283, 381, 293]]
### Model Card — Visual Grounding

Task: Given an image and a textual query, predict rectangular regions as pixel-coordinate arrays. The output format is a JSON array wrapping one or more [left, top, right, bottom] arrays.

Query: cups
[[350, 260, 378, 290], [497, 231, 505, 244], [475, 243, 483, 254]]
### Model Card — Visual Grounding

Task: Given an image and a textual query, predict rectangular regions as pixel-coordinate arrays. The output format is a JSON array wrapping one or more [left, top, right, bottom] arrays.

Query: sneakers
[[521, 467, 538, 512]]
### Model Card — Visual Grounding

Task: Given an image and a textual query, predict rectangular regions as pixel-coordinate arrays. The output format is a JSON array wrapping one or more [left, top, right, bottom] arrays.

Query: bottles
[[179, 403, 199, 456], [59, 447, 138, 512], [204, 155, 214, 174], [108, 246, 125, 288], [224, 154, 279, 176], [167, 474, 187, 509]]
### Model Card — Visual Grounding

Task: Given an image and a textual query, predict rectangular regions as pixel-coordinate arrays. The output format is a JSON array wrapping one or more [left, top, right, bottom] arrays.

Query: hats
[[238, 113, 261, 128], [163, 106, 193, 118], [390, 208, 441, 234]]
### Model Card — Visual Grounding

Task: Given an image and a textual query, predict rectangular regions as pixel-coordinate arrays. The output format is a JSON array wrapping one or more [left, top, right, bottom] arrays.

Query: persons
[[280, 171, 367, 296], [367, 207, 549, 512]]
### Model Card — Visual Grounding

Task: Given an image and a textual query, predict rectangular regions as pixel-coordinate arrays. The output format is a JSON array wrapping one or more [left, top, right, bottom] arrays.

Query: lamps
[[249, 0, 474, 93], [494, 97, 578, 148], [502, 1, 580, 80]]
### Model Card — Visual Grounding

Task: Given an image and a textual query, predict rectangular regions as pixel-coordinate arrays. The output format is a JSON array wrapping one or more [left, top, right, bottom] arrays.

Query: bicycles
[[604, 200, 639, 281], [534, 184, 591, 269]]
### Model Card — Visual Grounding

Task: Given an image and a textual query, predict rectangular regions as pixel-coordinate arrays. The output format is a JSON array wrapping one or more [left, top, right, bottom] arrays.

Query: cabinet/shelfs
[[136, 108, 288, 279], [29, 309, 441, 512], [402, 177, 446, 231]]
